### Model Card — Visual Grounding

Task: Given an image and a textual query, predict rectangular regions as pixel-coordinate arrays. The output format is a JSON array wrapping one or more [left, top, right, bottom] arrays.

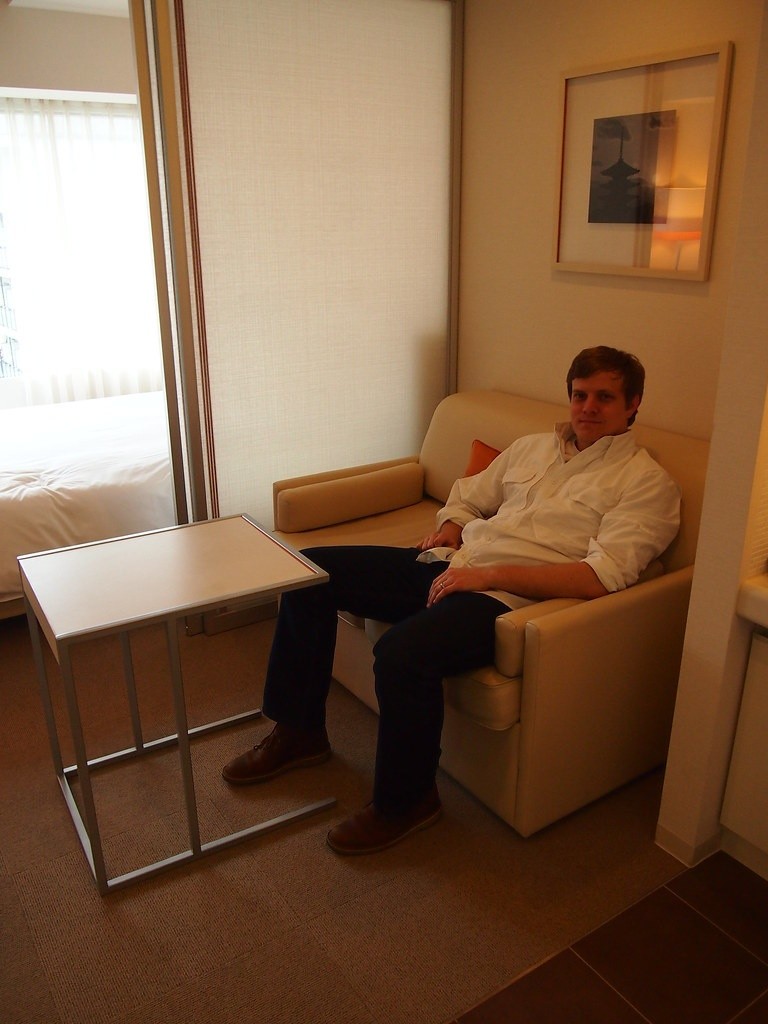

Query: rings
[[440, 583, 445, 588]]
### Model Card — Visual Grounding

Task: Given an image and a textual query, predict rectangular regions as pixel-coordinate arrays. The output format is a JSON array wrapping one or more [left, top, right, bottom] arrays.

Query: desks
[[15, 512, 337, 897]]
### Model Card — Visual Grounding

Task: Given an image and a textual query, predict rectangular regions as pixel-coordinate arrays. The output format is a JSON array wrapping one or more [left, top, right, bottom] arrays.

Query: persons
[[220, 345, 682, 857]]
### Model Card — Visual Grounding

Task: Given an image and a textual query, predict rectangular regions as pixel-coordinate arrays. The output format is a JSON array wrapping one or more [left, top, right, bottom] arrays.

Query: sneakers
[[221, 722, 334, 786], [327, 792, 442, 855]]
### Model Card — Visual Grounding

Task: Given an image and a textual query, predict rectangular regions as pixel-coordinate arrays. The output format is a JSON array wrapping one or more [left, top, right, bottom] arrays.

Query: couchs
[[265, 388, 711, 839]]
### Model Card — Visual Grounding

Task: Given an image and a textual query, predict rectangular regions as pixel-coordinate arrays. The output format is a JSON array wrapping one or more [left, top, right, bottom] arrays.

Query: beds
[[0, 391, 193, 623]]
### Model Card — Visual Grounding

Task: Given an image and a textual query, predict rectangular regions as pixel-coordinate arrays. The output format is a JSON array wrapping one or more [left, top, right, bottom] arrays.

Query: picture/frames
[[550, 39, 734, 283]]
